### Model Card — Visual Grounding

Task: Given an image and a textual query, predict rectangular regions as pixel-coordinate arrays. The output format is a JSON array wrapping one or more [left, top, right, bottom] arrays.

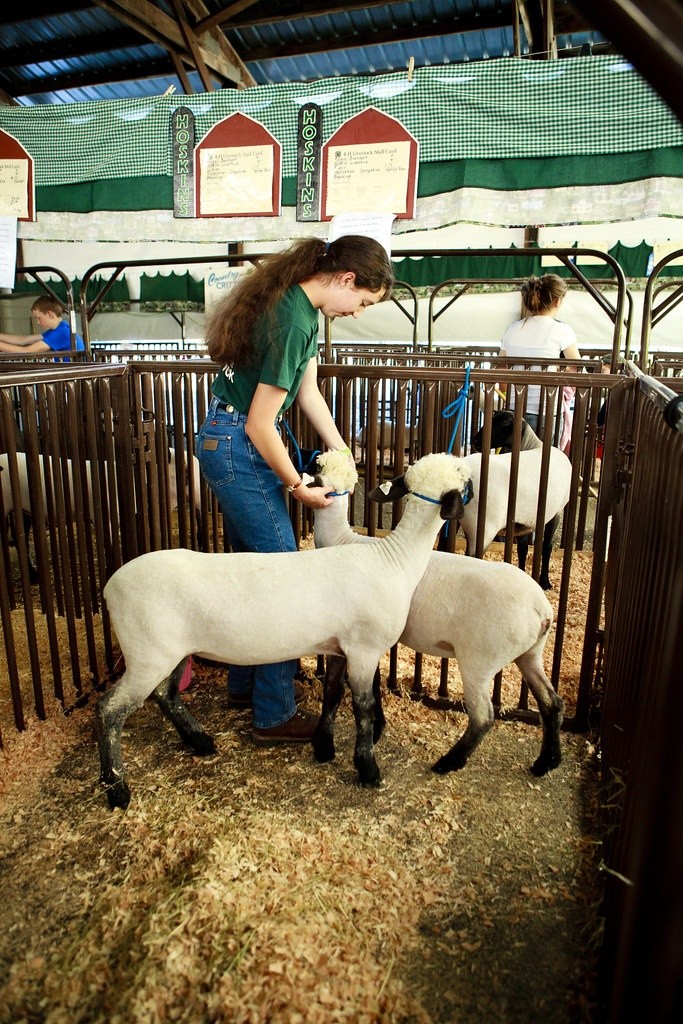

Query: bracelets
[[342, 448, 353, 456]]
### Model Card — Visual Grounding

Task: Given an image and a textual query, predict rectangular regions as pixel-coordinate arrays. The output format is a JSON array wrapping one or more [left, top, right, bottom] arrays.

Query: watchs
[[285, 473, 302, 493]]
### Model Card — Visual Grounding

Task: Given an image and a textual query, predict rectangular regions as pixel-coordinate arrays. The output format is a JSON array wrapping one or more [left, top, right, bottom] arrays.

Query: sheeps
[[356, 416, 419, 453], [290, 448, 563, 778], [0, 448, 206, 587], [458, 410, 573, 591], [94, 452, 475, 813]]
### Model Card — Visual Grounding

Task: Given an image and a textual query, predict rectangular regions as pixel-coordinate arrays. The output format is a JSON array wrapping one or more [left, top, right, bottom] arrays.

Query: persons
[[194, 236, 397, 744], [497, 275, 625, 448], [0, 296, 87, 363]]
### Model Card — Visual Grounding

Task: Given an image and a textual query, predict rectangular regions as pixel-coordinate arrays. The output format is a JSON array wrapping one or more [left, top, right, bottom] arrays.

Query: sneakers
[[228, 680, 306, 709], [251, 708, 321, 746]]
